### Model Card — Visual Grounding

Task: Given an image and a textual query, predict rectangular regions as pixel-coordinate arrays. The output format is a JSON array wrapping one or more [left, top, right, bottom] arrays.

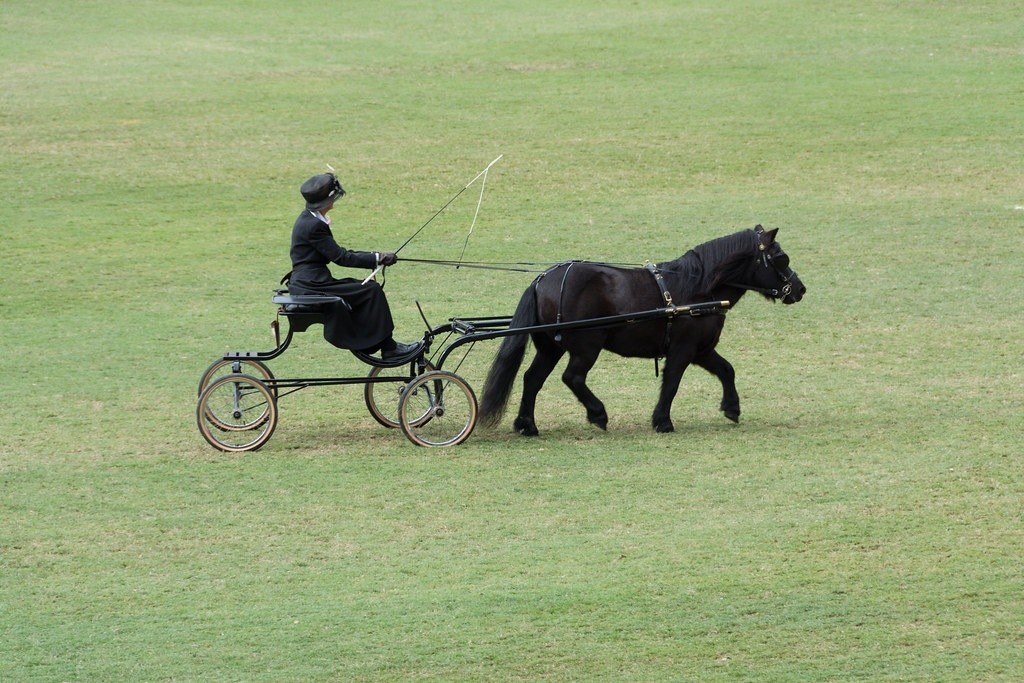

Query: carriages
[[195, 220, 807, 453]]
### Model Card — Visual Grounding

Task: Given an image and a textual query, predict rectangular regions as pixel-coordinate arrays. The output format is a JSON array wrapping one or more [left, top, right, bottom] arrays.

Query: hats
[[301, 173, 346, 211]]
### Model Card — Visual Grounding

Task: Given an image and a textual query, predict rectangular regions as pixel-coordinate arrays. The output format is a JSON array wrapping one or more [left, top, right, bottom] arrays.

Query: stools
[[272, 287, 349, 323]]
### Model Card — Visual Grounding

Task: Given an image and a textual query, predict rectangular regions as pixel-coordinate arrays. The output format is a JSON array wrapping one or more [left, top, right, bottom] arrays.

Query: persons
[[287, 173, 420, 362]]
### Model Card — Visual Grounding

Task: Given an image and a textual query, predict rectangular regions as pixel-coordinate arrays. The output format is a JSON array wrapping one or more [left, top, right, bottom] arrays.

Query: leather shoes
[[381, 342, 420, 360]]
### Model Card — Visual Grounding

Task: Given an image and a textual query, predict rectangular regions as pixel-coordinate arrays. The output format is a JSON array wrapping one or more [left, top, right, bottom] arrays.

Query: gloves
[[378, 252, 397, 266]]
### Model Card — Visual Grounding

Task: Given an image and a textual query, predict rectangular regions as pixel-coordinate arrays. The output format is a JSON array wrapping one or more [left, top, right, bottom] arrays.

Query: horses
[[476, 224, 806, 437]]
